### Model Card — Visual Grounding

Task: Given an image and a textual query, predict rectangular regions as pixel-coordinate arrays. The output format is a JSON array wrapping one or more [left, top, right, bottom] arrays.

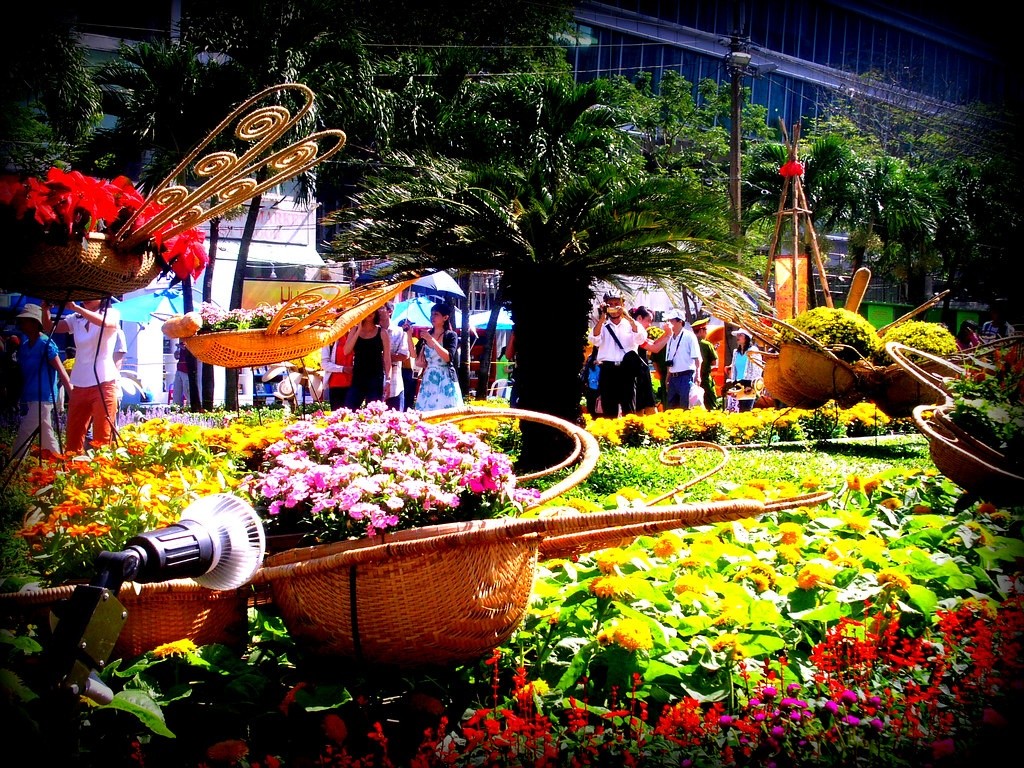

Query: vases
[[38, 578, 257, 669], [760, 358, 836, 411], [870, 362, 949, 420], [779, 343, 852, 402], [255, 517, 548, 671], [915, 398, 1018, 488], [10, 217, 154, 294]]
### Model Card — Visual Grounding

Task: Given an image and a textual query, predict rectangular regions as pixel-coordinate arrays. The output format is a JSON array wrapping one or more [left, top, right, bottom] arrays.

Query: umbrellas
[[356, 259, 468, 302], [390, 297, 466, 337], [471, 305, 515, 332]]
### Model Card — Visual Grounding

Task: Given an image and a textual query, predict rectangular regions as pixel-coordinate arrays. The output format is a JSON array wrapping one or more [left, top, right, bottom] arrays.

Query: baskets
[[17, 578, 244, 664], [914, 401, 1024, 505], [746, 344, 958, 418], [180, 326, 332, 372], [249, 404, 600, 663], [24, 226, 170, 302]]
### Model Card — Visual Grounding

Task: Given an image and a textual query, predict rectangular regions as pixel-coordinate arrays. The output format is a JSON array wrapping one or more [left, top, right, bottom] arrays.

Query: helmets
[[604, 289, 625, 303]]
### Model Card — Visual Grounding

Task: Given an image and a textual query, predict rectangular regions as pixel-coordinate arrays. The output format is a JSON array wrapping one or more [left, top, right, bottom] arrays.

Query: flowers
[[766, 306, 1024, 456], [198, 292, 340, 335], [33, 393, 536, 562], [0, 166, 206, 284]]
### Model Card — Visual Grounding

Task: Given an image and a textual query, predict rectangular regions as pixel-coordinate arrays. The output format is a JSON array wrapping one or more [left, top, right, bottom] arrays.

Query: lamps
[[32, 492, 269, 765], [266, 262, 278, 278]]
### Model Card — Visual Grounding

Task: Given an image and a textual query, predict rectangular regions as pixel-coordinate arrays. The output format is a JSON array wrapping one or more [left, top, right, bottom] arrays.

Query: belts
[[670, 370, 694, 377]]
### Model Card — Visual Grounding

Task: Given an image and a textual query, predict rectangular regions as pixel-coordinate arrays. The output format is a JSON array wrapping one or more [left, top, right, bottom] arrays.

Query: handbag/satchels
[[595, 396, 603, 414], [688, 381, 705, 410], [581, 355, 600, 389], [415, 340, 427, 367], [619, 350, 649, 378]]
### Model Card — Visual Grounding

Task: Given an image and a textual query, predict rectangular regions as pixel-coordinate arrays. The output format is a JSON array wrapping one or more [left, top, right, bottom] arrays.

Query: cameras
[[603, 304, 619, 313]]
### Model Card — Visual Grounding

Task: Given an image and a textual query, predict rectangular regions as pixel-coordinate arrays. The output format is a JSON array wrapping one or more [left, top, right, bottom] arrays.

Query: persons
[[584, 289, 764, 418], [977, 297, 1015, 354], [496, 344, 515, 396], [1, 296, 128, 458], [306, 299, 467, 410], [173, 341, 193, 412], [955, 320, 983, 360]]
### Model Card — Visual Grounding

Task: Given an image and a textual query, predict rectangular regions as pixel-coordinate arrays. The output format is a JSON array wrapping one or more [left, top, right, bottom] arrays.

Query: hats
[[690, 317, 709, 330], [14, 302, 43, 331], [732, 327, 753, 340], [661, 309, 686, 322]]
